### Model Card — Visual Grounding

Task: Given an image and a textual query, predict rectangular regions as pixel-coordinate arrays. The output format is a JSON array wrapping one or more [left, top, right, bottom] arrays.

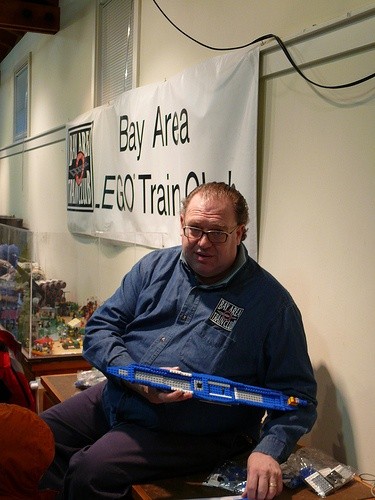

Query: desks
[[0, 317, 98, 397], [35, 372, 375, 500]]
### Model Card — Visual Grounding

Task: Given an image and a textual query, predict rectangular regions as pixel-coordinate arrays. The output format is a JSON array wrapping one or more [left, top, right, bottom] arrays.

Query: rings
[[268, 482, 277, 487]]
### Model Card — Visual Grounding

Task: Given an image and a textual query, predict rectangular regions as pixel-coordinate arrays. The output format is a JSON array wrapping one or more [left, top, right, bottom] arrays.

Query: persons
[[36, 181, 319, 500]]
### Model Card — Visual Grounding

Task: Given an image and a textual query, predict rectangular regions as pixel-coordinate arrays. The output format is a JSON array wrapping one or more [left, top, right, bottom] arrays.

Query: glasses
[[182, 212, 240, 244]]
